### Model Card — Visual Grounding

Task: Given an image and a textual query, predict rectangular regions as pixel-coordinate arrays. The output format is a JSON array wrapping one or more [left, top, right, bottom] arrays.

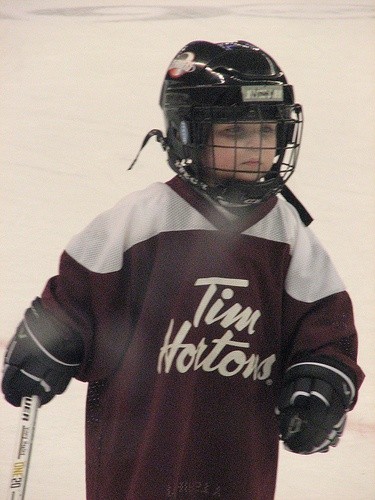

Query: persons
[[0, 39, 367, 500]]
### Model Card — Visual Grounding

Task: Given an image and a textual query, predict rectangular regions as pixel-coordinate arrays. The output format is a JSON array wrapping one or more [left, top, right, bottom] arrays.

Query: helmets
[[159, 41, 306, 205]]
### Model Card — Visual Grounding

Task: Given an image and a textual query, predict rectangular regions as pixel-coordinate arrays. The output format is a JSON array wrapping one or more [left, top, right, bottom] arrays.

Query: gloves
[[277, 356, 358, 455], [2, 298, 86, 406]]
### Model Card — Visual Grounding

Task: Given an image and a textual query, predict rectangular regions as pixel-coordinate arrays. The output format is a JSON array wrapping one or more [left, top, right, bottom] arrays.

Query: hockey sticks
[[10, 393, 39, 499]]
